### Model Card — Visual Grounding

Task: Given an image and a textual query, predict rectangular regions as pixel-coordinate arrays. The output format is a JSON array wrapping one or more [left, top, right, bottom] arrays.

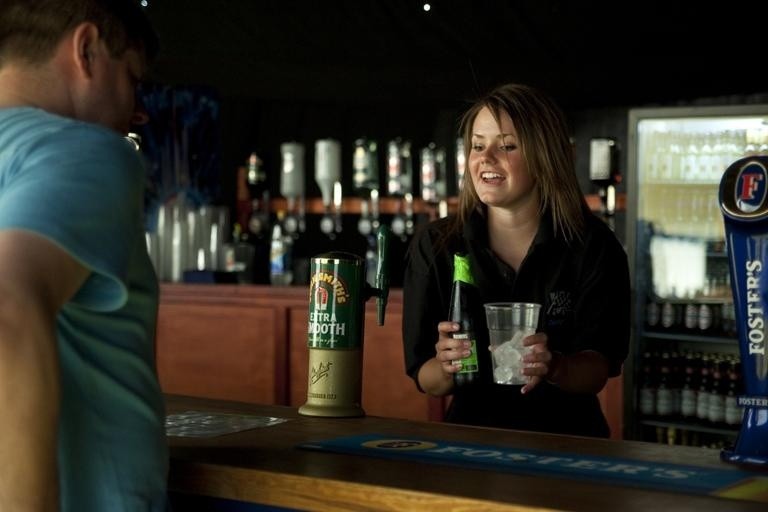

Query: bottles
[[636, 128, 768, 455], [242, 133, 476, 287], [446, 249, 485, 392], [588, 134, 620, 234]]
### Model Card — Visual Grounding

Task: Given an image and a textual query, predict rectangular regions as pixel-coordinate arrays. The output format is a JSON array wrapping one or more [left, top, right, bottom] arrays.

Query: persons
[[0, 0, 170, 512], [402, 84, 630, 438]]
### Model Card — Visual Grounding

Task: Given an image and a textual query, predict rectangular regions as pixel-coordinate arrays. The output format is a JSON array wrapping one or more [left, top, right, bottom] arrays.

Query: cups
[[483, 301, 543, 387]]
